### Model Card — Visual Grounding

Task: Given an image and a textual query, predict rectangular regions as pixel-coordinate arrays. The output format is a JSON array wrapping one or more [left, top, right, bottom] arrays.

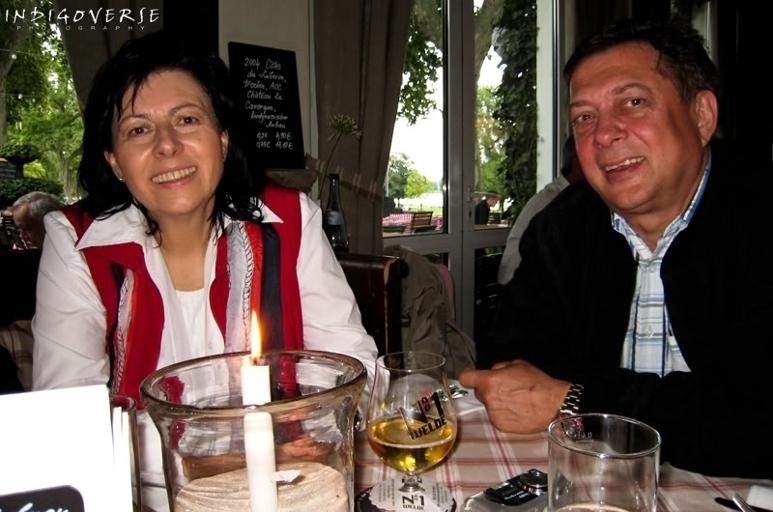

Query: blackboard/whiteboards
[[227, 41, 305, 171]]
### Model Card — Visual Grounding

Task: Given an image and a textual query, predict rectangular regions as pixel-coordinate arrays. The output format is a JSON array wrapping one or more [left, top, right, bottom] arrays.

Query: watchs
[[556, 380, 590, 442]]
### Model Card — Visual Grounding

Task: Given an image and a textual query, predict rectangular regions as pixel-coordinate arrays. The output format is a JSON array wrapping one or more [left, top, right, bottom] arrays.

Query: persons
[[10, 191, 66, 250], [475, 190, 499, 259], [494, 133, 586, 331], [457, 14, 773, 481], [29, 29, 391, 487]]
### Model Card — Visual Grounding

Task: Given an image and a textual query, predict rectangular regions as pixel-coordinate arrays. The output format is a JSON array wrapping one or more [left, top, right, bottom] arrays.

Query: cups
[[547, 413, 664, 512], [139, 348, 368, 512]]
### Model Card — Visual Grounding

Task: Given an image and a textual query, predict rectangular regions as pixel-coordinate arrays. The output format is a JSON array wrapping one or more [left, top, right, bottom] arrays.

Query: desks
[[354, 389, 773, 512]]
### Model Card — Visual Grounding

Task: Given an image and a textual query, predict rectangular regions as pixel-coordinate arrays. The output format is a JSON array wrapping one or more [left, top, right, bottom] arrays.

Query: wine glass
[[366, 347, 459, 493]]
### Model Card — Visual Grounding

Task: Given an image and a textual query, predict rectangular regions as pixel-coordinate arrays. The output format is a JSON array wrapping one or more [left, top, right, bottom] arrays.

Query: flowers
[[317, 113, 362, 200]]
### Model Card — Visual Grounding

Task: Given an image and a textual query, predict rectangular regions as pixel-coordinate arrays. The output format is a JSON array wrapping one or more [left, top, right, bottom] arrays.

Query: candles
[[242, 309, 277, 512]]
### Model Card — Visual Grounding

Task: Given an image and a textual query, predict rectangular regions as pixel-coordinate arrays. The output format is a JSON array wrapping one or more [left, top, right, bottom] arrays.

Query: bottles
[[323, 172, 350, 258]]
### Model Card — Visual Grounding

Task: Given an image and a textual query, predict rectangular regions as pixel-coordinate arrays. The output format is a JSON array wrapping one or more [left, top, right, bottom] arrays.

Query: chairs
[[0, 250, 410, 394], [382, 212, 436, 237]]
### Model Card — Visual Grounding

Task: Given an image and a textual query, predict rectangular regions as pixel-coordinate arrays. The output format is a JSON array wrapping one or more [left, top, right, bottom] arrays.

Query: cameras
[[464, 469, 571, 512]]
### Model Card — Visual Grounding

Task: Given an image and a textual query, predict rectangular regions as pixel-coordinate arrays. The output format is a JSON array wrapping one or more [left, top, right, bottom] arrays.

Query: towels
[[381, 374, 485, 416]]
